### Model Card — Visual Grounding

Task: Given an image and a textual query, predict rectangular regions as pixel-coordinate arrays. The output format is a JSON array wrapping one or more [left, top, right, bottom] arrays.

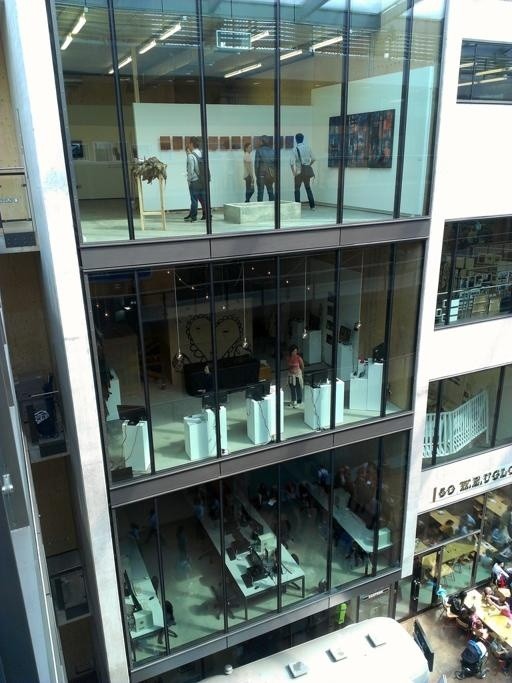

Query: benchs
[[223, 200, 302, 224]]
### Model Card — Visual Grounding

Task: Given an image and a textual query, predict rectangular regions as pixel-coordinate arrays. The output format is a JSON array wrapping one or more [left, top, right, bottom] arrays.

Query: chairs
[[159, 600, 177, 643], [491, 577, 510, 598], [440, 597, 512, 678]]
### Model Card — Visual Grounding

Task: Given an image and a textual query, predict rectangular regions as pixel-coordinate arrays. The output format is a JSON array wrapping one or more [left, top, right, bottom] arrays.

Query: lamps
[[309, 35, 343, 49], [60, 12, 87, 50], [280, 49, 301, 60], [223, 61, 262, 79], [107, 21, 182, 75], [249, 30, 269, 42]]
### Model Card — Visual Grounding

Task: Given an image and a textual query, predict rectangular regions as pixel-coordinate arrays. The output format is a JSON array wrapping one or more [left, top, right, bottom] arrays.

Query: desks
[[118, 534, 164, 640], [474, 493, 507, 517], [429, 508, 498, 557], [194, 463, 394, 620], [415, 537, 456, 588]]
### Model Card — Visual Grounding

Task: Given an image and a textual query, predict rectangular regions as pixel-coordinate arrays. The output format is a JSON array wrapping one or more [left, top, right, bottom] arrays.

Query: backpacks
[[191, 151, 210, 183]]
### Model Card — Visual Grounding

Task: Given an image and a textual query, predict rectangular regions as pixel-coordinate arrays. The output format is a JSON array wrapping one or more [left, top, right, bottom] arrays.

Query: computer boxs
[[231, 541, 250, 554], [247, 566, 267, 581], [131, 610, 153, 632]]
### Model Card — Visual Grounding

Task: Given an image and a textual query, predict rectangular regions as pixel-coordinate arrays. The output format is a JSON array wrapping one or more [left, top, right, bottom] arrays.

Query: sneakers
[[288, 401, 301, 409]]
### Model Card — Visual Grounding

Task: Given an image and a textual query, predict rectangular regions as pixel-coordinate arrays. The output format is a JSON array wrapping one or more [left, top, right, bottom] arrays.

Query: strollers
[[456, 636, 491, 679]]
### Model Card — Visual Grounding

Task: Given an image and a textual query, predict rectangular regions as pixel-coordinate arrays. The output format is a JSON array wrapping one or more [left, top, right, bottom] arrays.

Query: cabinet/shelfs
[[103, 367, 122, 421]]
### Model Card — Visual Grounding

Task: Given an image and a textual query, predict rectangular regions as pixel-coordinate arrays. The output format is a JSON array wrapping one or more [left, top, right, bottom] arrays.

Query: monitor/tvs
[[202, 392, 227, 409], [246, 382, 270, 400], [304, 368, 328, 388], [251, 549, 263, 574], [372, 342, 385, 363], [131, 592, 143, 614], [232, 528, 243, 547], [123, 569, 136, 596], [117, 405, 148, 425]]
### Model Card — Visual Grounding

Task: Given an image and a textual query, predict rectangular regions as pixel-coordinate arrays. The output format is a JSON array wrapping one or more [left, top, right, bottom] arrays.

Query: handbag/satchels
[[301, 165, 314, 179], [265, 168, 275, 183]]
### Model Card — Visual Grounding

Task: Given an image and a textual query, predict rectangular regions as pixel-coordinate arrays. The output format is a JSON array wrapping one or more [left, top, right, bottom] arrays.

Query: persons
[[184, 137, 212, 221], [242, 143, 255, 203], [448, 558, 512, 677], [291, 133, 316, 209], [286, 344, 304, 409], [481, 503, 512, 567], [184, 147, 199, 218], [417, 510, 475, 543], [256, 134, 275, 201]]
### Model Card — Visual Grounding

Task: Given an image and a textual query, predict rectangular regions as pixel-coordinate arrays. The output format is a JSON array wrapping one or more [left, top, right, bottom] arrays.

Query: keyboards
[[226, 547, 236, 561], [241, 574, 253, 588]]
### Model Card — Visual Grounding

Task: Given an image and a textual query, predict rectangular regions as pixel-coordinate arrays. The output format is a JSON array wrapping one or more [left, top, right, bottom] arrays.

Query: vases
[[101, 321, 142, 393]]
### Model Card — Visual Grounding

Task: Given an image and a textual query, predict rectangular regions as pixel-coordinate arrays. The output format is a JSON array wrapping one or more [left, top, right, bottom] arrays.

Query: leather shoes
[[184, 215, 206, 221]]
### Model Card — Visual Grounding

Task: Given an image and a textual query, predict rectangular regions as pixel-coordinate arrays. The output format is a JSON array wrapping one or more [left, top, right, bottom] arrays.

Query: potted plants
[[130, 155, 168, 184]]
